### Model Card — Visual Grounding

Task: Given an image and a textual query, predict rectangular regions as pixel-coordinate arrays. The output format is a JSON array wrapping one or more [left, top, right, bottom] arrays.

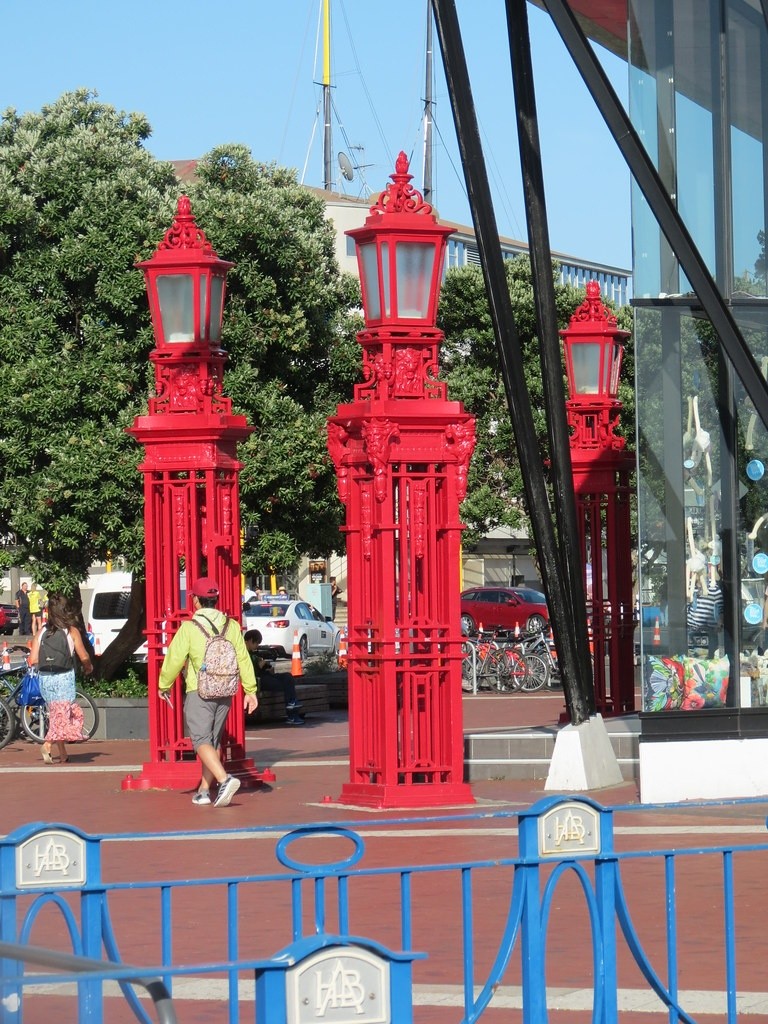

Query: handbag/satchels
[[20, 665, 45, 706]]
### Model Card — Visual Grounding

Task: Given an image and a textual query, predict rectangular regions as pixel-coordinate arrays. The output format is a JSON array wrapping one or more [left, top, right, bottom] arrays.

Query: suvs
[[0, 603, 19, 637]]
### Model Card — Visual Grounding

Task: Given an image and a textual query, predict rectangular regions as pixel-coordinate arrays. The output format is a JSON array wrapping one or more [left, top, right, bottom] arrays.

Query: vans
[[87, 572, 228, 682]]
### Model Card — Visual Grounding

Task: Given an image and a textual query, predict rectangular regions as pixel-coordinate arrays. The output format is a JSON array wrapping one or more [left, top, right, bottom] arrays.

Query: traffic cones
[[288, 629, 302, 678], [653, 616, 663, 645], [338, 626, 350, 671], [584, 617, 596, 653]]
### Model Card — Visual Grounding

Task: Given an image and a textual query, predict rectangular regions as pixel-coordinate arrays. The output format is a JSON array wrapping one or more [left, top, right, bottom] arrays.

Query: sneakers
[[285, 715, 306, 724], [286, 700, 304, 710], [192, 789, 212, 805], [213, 773, 241, 808]]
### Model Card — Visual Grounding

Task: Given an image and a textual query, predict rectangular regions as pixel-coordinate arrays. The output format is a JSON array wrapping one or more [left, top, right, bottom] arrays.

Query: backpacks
[[38, 629, 75, 671], [190, 615, 240, 699]]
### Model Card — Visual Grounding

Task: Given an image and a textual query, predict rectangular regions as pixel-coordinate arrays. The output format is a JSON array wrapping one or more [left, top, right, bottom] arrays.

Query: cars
[[250, 598, 342, 655], [458, 585, 553, 638]]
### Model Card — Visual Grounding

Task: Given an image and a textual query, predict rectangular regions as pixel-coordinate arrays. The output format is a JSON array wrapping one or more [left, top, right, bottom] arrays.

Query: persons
[[504, 593, 512, 604], [155, 579, 258, 809], [16, 583, 33, 638], [254, 586, 266, 602], [26, 583, 44, 638], [310, 562, 326, 585], [752, 536, 768, 580], [330, 578, 342, 622], [244, 583, 257, 603], [278, 587, 288, 596], [759, 586, 768, 632], [656, 576, 669, 630], [242, 631, 306, 726], [29, 594, 95, 766]]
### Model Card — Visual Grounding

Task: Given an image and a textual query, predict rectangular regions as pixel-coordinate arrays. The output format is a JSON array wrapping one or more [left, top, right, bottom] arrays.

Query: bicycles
[[0, 645, 104, 752], [443, 615, 593, 696]]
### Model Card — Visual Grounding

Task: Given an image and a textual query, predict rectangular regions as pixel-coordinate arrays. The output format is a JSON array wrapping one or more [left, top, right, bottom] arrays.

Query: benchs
[[256, 676, 329, 725]]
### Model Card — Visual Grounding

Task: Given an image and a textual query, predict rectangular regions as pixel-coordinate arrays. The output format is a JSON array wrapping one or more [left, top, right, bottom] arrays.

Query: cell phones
[[163, 693, 173, 709]]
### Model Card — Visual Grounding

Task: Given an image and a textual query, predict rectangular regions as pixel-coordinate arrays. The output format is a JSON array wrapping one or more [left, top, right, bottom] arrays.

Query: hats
[[188, 577, 219, 597]]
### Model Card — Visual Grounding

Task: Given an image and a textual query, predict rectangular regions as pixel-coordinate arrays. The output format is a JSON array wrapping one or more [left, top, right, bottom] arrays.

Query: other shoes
[[42, 746, 53, 764]]
